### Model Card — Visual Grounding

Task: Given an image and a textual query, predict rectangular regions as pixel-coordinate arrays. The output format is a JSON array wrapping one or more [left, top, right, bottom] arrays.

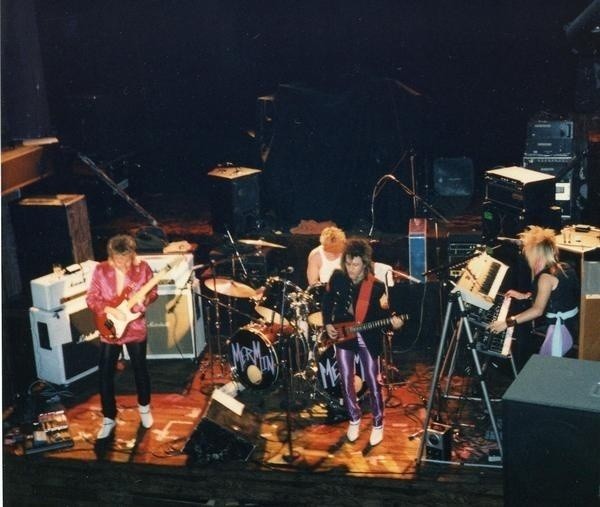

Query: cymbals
[[249, 286, 265, 300], [239, 240, 284, 248], [205, 278, 254, 297], [310, 312, 323, 327], [256, 306, 287, 325]]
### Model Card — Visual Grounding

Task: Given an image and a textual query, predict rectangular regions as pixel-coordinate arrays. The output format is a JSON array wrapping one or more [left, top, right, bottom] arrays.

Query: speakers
[[8, 194, 95, 298], [481, 165, 560, 245], [208, 166, 263, 240], [181, 387, 257, 466], [425, 421, 452, 466], [29, 293, 101, 387], [501, 354, 600, 507], [147, 280, 207, 359]]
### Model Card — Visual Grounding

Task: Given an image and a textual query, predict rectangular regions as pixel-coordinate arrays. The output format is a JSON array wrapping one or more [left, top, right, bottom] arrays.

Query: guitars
[[97, 254, 186, 340], [320, 310, 410, 353]]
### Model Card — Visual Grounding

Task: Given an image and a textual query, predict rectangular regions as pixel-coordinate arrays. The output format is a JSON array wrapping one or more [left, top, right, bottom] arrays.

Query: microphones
[[497, 236, 522, 251], [368, 225, 376, 237], [167, 292, 182, 314], [279, 266, 293, 278]]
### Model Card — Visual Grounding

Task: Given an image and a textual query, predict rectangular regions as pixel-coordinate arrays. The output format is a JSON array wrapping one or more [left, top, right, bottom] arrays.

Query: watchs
[[509, 316, 516, 324]]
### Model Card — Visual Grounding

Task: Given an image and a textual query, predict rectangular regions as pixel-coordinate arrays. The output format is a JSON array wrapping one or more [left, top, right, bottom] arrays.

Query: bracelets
[[505, 318, 512, 327]]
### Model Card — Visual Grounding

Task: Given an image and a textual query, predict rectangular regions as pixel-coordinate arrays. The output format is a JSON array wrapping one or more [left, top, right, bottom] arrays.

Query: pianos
[[454, 253, 515, 357]]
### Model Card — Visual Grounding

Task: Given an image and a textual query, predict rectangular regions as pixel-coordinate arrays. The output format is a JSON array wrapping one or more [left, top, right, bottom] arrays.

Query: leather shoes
[[347, 417, 360, 442], [97, 417, 117, 439], [137, 404, 154, 428], [369, 423, 384, 446]]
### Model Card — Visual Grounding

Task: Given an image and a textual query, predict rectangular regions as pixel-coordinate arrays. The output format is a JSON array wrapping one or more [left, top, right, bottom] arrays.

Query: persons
[[320, 238, 404, 445], [85, 235, 158, 440], [488, 225, 579, 360], [306, 224, 350, 288]]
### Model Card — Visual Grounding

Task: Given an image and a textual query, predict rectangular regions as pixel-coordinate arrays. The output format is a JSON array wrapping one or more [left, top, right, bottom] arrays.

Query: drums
[[254, 276, 303, 327], [226, 321, 309, 391], [305, 283, 328, 326]]
